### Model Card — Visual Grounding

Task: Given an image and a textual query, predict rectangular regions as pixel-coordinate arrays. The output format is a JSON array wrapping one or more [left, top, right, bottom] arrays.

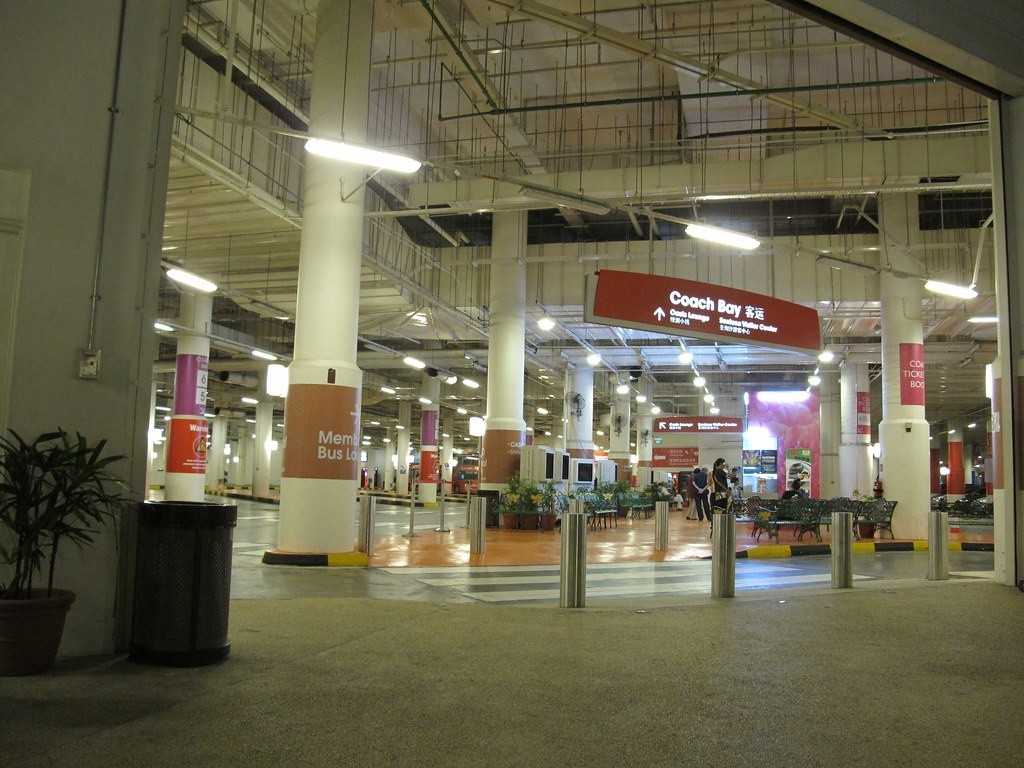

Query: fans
[[564, 391, 585, 416]]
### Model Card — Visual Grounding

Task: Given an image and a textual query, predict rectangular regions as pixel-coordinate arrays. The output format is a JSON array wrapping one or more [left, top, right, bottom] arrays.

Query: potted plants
[[0, 427, 137, 678]]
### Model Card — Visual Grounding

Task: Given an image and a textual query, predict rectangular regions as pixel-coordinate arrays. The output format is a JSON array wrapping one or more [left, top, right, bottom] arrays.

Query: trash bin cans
[[129, 499, 238, 665]]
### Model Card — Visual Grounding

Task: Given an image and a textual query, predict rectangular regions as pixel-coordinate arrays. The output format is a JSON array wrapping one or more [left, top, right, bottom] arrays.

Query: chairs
[[745, 496, 898, 543]]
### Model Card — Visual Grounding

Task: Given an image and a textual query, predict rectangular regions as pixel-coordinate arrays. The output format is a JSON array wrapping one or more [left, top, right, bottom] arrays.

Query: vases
[[503, 507, 628, 531]]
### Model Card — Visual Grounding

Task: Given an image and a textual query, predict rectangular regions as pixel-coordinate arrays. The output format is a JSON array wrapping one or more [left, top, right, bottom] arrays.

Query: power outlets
[[570, 411, 574, 415]]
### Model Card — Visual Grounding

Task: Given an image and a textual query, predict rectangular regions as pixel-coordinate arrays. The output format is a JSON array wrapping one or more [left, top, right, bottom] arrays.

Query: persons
[[712, 457, 734, 513], [873, 476, 884, 499], [686, 467, 740, 521], [781, 478, 802, 500], [657, 481, 685, 512]]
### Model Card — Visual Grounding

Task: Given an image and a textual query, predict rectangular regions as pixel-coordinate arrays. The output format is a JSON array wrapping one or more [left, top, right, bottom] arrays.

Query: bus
[[451, 451, 480, 494], [408, 463, 442, 493]]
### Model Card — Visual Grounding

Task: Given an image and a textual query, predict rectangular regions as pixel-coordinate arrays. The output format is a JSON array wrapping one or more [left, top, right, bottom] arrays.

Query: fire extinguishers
[[873, 473, 883, 498]]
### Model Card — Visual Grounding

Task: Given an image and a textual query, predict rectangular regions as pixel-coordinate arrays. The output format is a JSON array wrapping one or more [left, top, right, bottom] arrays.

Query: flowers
[[491, 476, 630, 512]]
